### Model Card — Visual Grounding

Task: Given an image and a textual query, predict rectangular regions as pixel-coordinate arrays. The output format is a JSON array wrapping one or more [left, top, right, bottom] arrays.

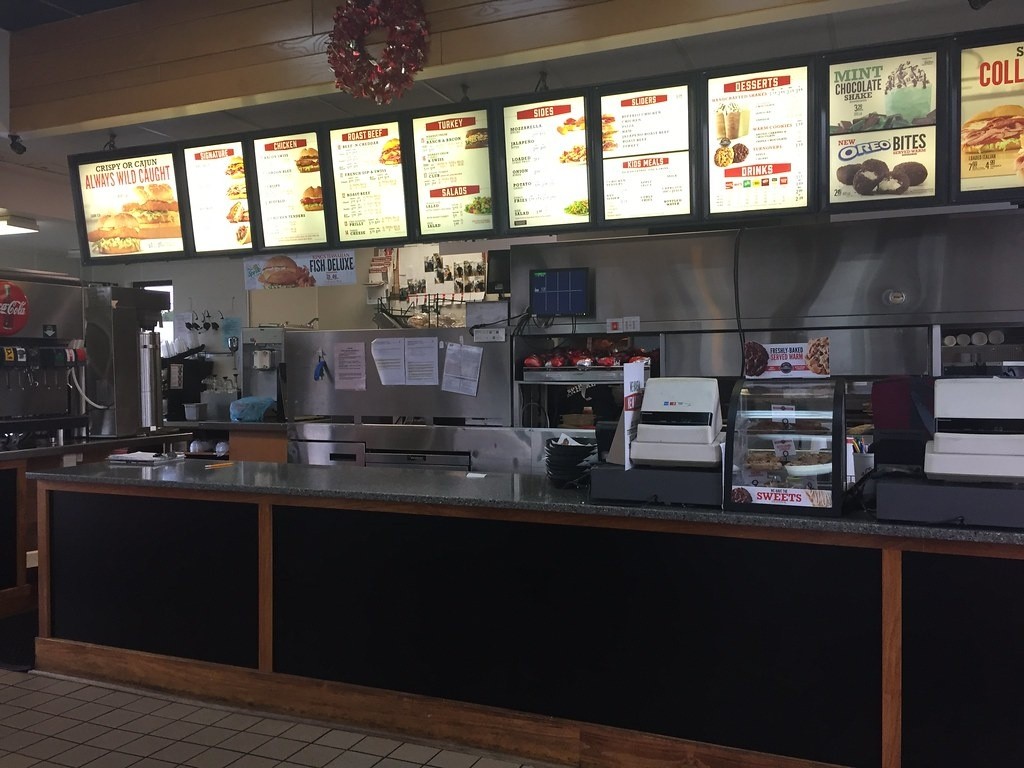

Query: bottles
[[3, 285, 14, 329]]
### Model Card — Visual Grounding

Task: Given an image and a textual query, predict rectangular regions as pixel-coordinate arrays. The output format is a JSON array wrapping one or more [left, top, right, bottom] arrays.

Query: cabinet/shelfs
[[165, 427, 289, 464], [724, 377, 874, 516]]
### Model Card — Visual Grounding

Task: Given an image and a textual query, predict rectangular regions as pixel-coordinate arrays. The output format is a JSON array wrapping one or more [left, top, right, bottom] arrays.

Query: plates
[[785, 462, 833, 477], [544, 436, 598, 488]]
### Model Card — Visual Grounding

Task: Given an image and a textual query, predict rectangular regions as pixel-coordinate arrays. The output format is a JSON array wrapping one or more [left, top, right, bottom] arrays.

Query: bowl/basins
[[189, 440, 203, 453], [216, 442, 228, 452]]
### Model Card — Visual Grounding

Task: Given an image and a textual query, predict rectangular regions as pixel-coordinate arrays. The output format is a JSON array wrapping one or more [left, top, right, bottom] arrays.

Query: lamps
[[8, 134, 27, 154]]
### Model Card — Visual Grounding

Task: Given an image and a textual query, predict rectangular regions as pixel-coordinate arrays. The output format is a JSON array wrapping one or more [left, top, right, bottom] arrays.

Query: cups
[[161, 330, 199, 358], [725, 111, 740, 139], [853, 453, 875, 482], [739, 110, 750, 137], [714, 112, 726, 139]]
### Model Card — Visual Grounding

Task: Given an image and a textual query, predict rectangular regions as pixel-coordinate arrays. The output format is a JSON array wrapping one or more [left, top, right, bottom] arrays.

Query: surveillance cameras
[[11, 142, 26, 155]]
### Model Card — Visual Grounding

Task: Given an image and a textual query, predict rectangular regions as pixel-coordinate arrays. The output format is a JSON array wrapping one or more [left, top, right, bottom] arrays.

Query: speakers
[[486, 250, 511, 294]]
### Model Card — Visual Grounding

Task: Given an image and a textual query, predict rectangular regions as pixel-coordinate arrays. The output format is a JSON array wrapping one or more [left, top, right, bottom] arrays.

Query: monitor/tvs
[[529, 266, 590, 318]]
